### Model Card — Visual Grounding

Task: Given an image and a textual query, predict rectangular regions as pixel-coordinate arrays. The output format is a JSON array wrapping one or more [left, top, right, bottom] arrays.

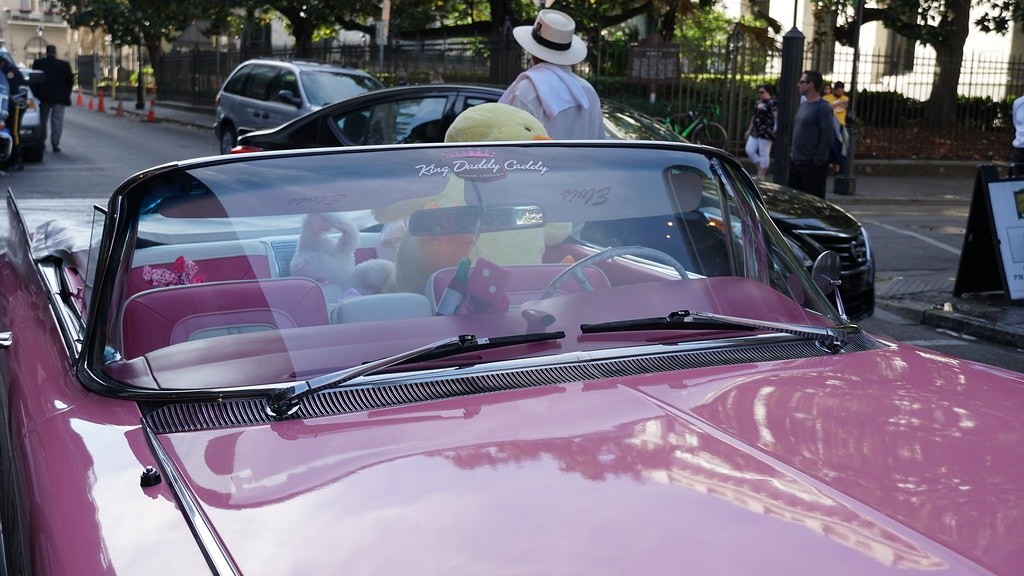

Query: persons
[[498, 10, 605, 240], [744, 84, 778, 180], [787, 71, 834, 201], [1012, 95, 1024, 178], [797, 80, 850, 175], [0, 55, 28, 173], [29, 44, 74, 153]]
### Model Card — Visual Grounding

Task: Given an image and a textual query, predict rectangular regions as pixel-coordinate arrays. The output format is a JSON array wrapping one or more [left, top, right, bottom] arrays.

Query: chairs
[[425, 260, 609, 317], [117, 277, 331, 366]]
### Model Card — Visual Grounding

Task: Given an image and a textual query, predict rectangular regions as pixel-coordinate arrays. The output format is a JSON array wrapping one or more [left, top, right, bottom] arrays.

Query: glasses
[[757, 91, 767, 93], [799, 80, 810, 84]]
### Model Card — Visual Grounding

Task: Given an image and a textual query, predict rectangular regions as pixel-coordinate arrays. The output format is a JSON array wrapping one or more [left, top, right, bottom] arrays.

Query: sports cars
[[2, 140, 1024, 575]]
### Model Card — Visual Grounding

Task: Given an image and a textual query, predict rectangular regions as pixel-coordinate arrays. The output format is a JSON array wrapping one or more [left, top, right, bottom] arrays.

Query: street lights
[[36, 27, 45, 59]]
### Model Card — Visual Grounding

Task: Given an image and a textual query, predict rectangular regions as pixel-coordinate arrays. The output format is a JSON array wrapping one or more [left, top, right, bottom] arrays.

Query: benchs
[[121, 231, 543, 299]]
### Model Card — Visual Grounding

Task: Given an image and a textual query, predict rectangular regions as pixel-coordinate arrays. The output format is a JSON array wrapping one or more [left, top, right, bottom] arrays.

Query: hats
[[512, 9, 588, 65]]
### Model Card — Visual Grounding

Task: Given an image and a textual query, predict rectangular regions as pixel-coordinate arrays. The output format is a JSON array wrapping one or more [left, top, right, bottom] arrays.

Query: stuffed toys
[[289, 103, 573, 317]]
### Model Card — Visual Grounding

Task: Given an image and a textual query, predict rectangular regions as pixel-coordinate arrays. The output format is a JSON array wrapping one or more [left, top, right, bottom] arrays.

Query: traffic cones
[[88, 97, 94, 109], [143, 99, 159, 123], [114, 99, 126, 117], [94, 89, 106, 113], [75, 86, 85, 106]]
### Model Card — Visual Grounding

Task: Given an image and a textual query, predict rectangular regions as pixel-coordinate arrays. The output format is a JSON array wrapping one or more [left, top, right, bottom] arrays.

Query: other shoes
[[53, 147, 60, 151]]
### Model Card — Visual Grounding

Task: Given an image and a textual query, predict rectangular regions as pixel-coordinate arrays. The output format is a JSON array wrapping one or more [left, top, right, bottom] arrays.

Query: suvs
[[213, 55, 402, 156]]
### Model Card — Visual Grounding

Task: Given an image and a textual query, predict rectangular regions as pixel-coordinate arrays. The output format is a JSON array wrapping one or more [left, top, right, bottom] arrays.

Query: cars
[[230, 81, 880, 322], [0, 48, 42, 169]]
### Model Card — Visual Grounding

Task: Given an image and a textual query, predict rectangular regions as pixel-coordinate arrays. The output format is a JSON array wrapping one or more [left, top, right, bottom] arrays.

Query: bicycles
[[651, 103, 727, 150]]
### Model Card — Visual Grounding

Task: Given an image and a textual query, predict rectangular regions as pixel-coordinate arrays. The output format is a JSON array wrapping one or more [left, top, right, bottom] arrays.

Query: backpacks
[[814, 99, 850, 164]]
